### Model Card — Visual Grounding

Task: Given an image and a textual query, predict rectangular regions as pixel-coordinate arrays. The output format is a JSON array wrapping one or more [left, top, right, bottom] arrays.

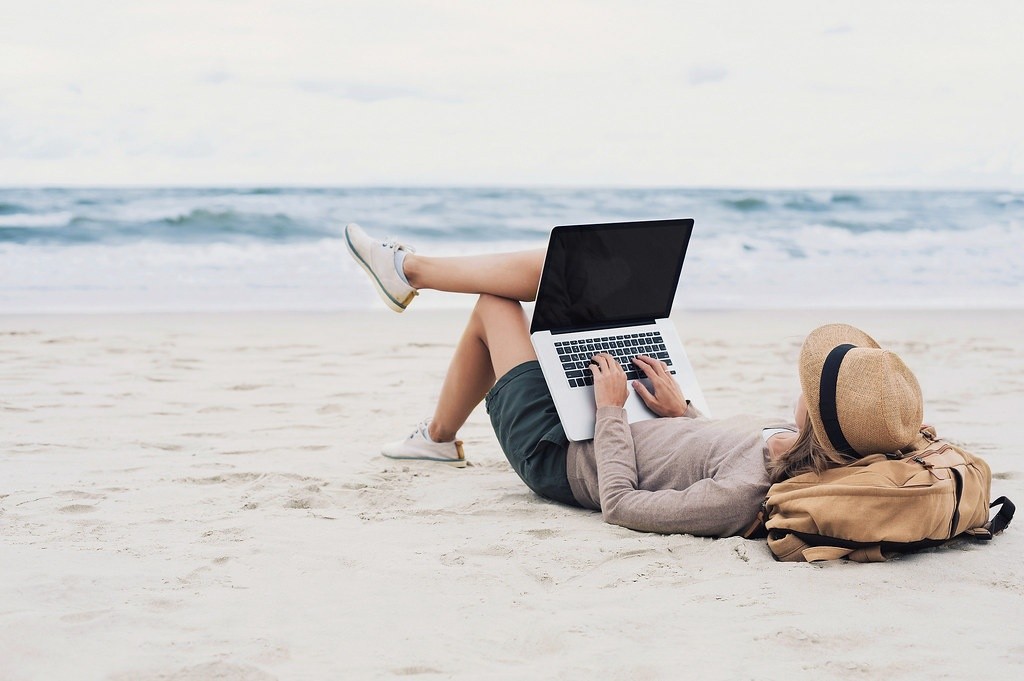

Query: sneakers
[[343, 222, 419, 313], [381, 417, 467, 467]]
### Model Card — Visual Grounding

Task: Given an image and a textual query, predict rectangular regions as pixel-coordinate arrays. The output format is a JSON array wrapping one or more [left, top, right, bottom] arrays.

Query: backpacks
[[764, 426, 1015, 564]]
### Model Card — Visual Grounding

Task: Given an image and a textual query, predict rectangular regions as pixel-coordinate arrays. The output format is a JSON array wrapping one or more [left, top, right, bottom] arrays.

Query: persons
[[344, 219, 924, 537]]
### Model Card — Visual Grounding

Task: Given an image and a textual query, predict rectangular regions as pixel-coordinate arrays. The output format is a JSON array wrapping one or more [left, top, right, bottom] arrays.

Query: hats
[[800, 324, 923, 466]]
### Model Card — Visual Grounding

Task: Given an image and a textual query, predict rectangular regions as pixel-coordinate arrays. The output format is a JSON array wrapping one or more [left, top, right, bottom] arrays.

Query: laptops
[[530, 218, 708, 442]]
